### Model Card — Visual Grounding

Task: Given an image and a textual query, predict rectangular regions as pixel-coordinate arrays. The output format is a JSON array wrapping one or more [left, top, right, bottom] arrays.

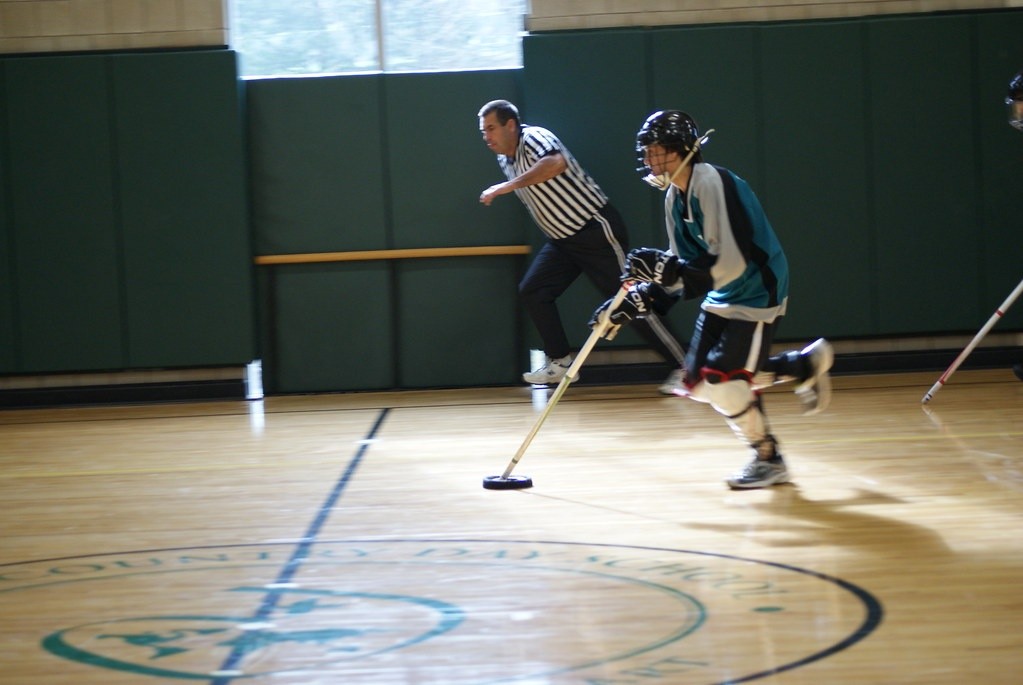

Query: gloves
[[620, 246, 679, 286], [588, 296, 638, 340]]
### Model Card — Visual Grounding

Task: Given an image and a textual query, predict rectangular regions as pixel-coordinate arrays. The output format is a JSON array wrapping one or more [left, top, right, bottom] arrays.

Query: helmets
[[635, 110, 702, 191]]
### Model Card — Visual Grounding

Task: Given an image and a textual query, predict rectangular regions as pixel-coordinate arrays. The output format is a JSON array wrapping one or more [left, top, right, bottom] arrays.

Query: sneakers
[[726, 452, 790, 489], [522, 355, 580, 387], [659, 368, 695, 396], [795, 338, 835, 416]]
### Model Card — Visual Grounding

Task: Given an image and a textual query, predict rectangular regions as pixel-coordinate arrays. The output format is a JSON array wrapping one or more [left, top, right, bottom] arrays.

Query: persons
[[589, 111, 833, 491], [475, 99, 688, 396], [1005, 77, 1023, 385]]
